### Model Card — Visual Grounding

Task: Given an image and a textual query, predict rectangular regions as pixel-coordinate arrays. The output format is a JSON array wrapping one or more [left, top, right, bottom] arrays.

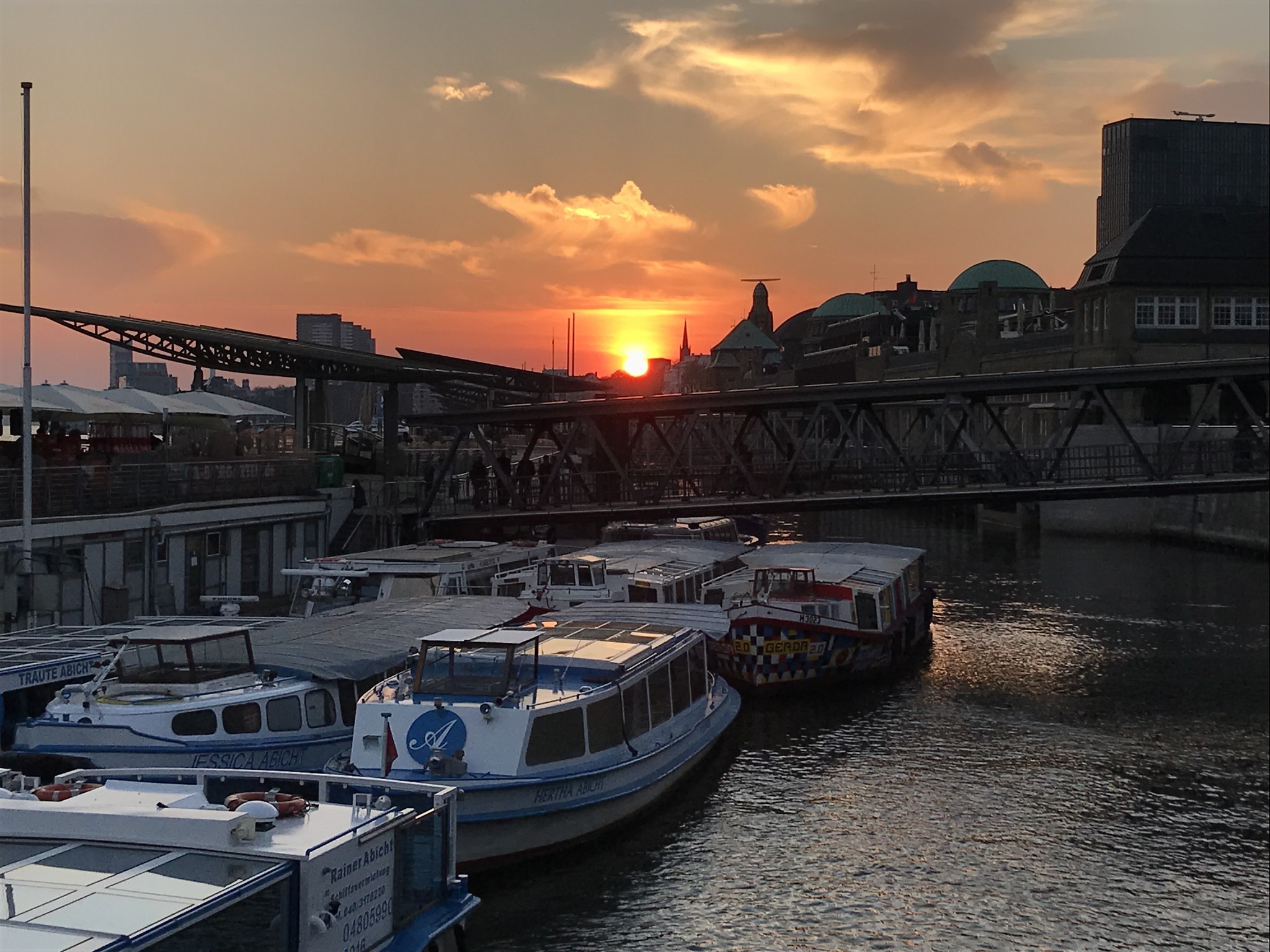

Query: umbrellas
[[0, 386, 293, 418]]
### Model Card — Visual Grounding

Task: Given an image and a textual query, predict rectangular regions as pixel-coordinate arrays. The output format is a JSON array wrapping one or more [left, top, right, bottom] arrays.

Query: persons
[[236, 415, 252, 455], [397, 431, 413, 446], [468, 453, 561, 509]]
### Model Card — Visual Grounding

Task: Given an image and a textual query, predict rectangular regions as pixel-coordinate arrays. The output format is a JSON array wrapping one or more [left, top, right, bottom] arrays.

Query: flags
[[385, 722, 399, 777]]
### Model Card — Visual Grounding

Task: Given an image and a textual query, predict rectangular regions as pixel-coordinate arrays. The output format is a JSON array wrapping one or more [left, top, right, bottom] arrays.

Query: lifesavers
[[429, 538, 455, 544], [316, 557, 338, 561], [225, 790, 308, 816], [35, 782, 103, 801], [512, 539, 536, 547]]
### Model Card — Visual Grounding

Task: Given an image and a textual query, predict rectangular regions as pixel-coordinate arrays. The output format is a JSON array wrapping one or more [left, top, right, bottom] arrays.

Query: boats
[[690, 540, 939, 697], [595, 510, 760, 550], [320, 600, 747, 875], [345, 417, 409, 435], [0, 592, 539, 778], [281, 539, 557, 617], [0, 761, 485, 952], [490, 534, 758, 610]]
